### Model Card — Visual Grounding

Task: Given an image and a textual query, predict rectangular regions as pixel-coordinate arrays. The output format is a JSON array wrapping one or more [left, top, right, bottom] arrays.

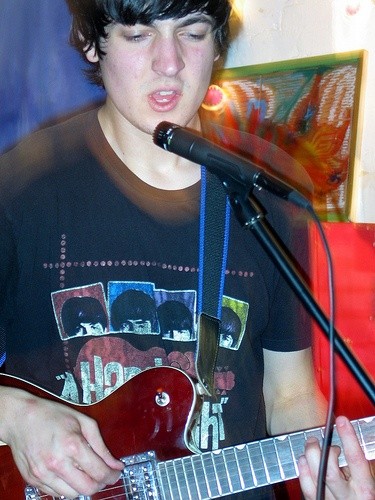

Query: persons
[[0, 0, 375, 500]]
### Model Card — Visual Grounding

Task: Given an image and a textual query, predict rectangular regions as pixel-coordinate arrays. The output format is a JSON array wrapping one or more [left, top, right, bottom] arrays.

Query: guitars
[[0, 366, 375, 500]]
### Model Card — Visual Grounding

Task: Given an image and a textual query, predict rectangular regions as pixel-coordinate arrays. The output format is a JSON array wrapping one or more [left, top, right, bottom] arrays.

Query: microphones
[[152, 121, 310, 208]]
